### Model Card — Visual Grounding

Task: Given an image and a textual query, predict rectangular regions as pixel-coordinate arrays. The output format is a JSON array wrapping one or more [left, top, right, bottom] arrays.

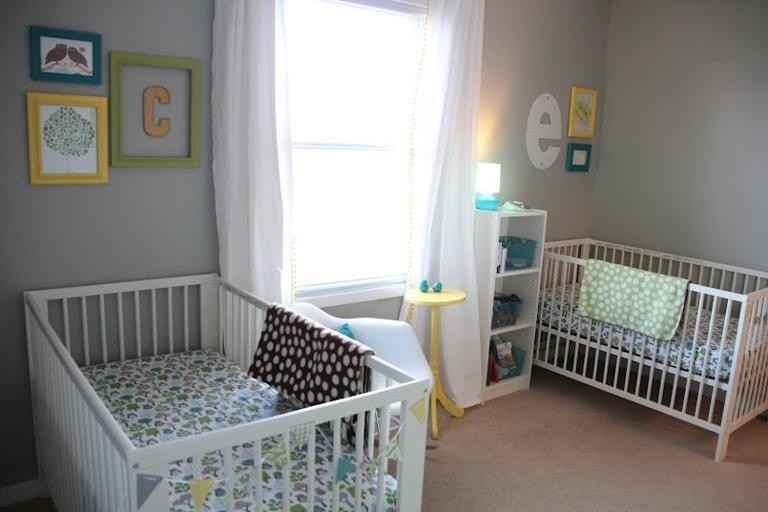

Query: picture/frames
[[110, 51, 200, 169], [26, 91, 108, 186], [29, 25, 102, 87], [567, 86, 598, 139], [566, 143, 591, 172]]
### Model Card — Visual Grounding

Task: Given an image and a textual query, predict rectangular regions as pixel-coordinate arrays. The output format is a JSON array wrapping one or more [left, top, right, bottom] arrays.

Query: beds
[[533, 239, 767, 462], [23, 273, 429, 512]]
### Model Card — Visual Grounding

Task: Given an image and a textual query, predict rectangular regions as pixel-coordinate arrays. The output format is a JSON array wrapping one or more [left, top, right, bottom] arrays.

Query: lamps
[[474, 162, 501, 210]]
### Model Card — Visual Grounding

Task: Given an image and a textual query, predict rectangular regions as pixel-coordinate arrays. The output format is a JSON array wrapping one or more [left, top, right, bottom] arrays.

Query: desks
[[403, 287, 466, 439]]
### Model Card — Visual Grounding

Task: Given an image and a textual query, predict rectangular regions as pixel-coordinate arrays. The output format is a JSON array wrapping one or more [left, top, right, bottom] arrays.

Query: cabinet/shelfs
[[474, 207, 548, 405]]
